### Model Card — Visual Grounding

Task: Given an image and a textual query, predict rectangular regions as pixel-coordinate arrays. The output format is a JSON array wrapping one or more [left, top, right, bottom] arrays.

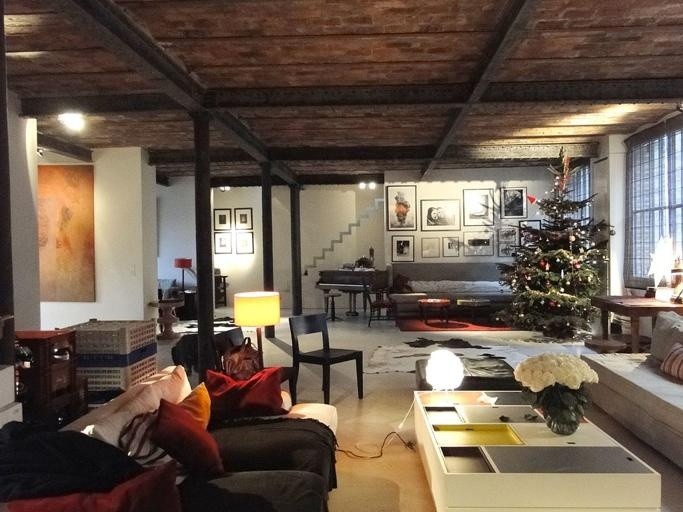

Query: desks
[[215, 275, 228, 309], [593, 292, 683, 355], [149, 299, 184, 340]]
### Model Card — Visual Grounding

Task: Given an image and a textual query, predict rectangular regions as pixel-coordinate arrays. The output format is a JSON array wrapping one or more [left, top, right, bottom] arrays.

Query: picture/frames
[[213, 208, 232, 230], [237, 232, 254, 255], [386, 185, 542, 261], [234, 208, 253, 230], [214, 232, 233, 254]]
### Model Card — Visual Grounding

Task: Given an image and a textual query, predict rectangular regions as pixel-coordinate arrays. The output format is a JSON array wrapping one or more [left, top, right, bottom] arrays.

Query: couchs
[[389, 263, 531, 319], [158, 281, 175, 299]]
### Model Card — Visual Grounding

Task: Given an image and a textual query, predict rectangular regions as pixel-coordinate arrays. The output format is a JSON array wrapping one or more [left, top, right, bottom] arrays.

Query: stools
[[326, 291, 342, 322], [457, 299, 491, 324], [421, 296, 449, 325]]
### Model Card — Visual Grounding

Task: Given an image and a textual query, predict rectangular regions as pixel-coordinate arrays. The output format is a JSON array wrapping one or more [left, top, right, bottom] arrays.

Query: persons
[[436, 207, 447, 226], [427, 207, 437, 226]]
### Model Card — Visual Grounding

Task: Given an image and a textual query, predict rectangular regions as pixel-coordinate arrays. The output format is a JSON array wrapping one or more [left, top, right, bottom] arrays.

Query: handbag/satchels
[[221, 336, 260, 380]]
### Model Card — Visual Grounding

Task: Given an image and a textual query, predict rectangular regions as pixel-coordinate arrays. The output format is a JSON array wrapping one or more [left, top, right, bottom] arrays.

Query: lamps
[[236, 291, 281, 370], [175, 258, 191, 294]]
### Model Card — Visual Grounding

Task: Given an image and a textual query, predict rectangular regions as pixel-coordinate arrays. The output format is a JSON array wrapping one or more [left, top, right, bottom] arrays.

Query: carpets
[[398, 321, 527, 331]]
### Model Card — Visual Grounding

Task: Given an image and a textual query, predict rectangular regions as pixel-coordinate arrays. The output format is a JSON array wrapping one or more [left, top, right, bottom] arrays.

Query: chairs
[[360, 276, 392, 327], [290, 317, 362, 405]]
